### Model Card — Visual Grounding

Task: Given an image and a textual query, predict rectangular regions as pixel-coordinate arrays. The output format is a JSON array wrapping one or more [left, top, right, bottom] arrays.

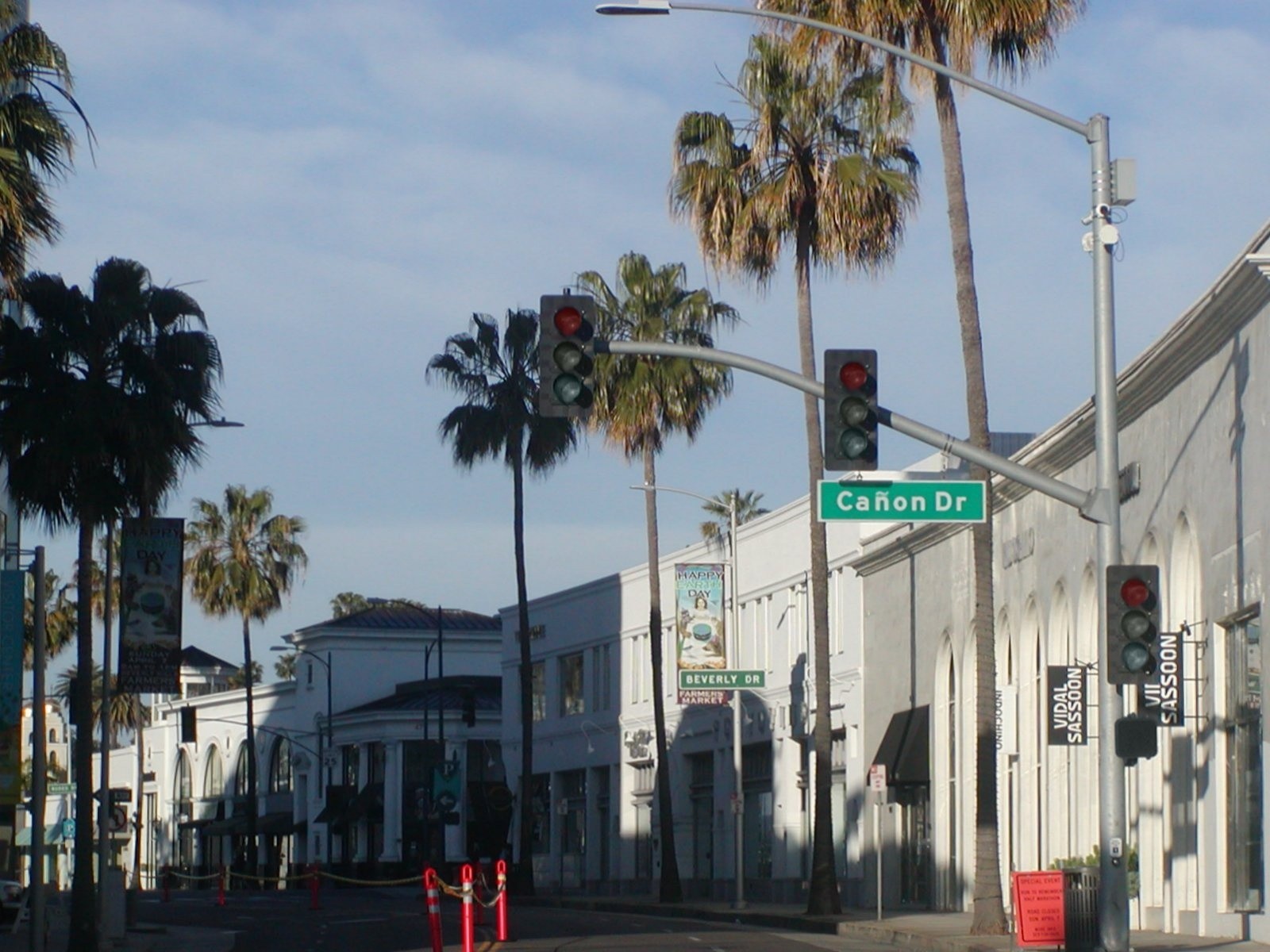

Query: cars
[[0, 881, 23, 903]]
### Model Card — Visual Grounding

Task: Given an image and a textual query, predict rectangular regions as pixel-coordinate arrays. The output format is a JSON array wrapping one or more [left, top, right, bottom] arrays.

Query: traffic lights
[[457, 685, 478, 726], [1114, 719, 1157, 758], [539, 296, 603, 418], [824, 350, 879, 471], [1106, 564, 1162, 684]]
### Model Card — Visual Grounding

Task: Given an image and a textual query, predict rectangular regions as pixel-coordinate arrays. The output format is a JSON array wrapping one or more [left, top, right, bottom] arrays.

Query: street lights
[[271, 646, 333, 883], [365, 595, 447, 775], [595, 0, 1130, 952], [628, 482, 752, 912]]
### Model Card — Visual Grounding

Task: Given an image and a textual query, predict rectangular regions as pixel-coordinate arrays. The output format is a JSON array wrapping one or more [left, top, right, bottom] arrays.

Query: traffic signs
[[92, 789, 131, 802]]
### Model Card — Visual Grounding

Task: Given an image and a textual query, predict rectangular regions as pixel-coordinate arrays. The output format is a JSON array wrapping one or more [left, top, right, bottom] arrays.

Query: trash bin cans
[[1062, 866, 1100, 952]]
